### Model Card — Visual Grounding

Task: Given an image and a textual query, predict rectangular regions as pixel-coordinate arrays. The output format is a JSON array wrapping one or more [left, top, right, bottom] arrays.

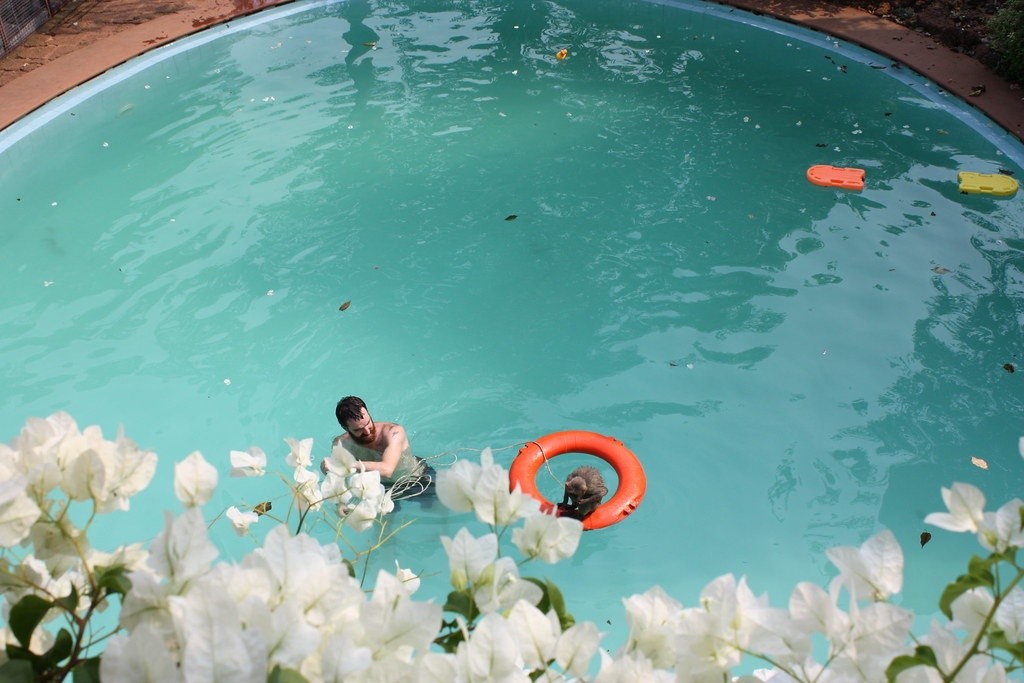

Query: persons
[[319, 394, 436, 518]]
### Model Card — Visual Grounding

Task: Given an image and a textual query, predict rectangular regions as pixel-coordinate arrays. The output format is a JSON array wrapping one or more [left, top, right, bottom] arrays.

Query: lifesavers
[[506, 430, 647, 529]]
